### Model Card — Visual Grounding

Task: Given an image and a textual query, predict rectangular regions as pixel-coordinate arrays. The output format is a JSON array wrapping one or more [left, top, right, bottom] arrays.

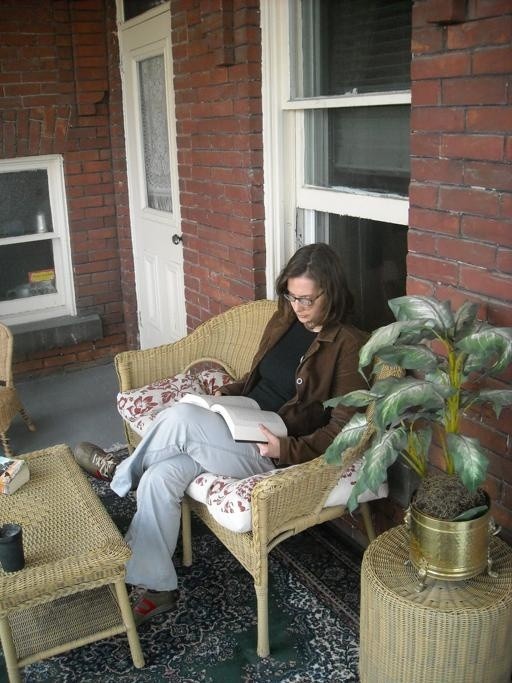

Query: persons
[[73, 239, 371, 635]]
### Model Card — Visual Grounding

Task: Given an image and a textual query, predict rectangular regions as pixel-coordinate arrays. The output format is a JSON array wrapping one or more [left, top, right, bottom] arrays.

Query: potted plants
[[323, 293, 512, 593]]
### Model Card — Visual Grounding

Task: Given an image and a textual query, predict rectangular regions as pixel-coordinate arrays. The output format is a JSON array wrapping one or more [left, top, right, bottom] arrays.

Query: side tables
[[358, 521, 511, 683]]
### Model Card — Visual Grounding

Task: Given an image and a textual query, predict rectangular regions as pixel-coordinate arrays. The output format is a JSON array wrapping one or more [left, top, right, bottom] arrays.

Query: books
[[0, 456, 31, 496], [177, 393, 288, 444]]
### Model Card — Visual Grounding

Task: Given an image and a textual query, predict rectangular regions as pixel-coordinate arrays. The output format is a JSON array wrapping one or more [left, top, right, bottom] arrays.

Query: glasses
[[283, 289, 327, 307]]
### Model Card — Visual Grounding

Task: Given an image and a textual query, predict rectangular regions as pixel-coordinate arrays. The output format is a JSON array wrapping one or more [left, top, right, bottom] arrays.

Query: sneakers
[[128, 587, 177, 628], [73, 440, 140, 492]]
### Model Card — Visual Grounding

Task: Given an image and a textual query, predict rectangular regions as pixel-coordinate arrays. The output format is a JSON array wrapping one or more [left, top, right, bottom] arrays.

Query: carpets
[[1, 439, 366, 683]]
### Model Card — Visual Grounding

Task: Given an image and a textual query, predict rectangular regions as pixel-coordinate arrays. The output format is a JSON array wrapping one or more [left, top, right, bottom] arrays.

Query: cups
[[0, 523, 26, 573]]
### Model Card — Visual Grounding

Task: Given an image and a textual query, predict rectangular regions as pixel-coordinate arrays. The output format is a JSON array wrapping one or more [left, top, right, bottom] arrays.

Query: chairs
[[0, 325, 35, 456], [115, 299, 376, 659]]
